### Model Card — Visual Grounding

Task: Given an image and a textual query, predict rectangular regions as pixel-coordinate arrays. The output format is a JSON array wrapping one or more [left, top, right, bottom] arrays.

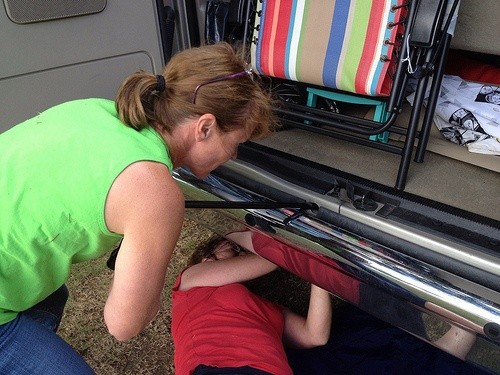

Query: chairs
[[205, 0, 460, 191]]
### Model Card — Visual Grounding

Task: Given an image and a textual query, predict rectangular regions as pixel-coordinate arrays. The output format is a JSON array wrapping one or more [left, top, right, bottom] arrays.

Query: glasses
[[192, 62, 261, 105]]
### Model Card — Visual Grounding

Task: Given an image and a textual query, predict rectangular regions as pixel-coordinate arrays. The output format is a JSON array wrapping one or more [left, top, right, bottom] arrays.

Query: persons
[[0, 41, 281, 375], [171, 231, 332, 375]]
